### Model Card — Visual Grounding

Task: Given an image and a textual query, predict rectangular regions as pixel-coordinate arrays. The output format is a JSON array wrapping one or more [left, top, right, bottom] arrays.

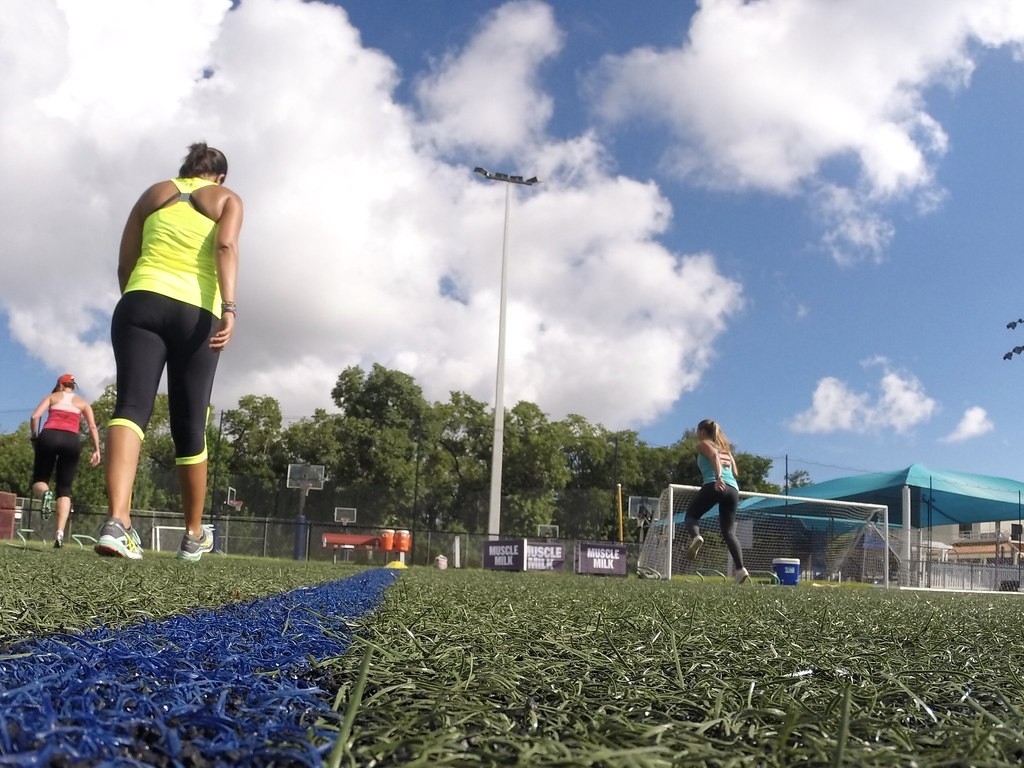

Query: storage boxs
[[772, 558, 802, 586]]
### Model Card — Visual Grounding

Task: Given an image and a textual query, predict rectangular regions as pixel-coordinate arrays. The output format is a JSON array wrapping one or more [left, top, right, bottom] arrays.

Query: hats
[[51, 374, 74, 393]]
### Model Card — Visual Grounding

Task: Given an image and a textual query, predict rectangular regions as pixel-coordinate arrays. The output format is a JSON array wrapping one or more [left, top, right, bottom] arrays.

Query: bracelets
[[31, 436, 37, 441], [715, 474, 722, 480], [220, 302, 242, 322]]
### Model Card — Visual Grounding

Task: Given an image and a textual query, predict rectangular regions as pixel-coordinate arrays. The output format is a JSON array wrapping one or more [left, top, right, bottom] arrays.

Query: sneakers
[[93, 517, 143, 560], [177, 524, 215, 562], [687, 536, 704, 563], [734, 567, 749, 586]]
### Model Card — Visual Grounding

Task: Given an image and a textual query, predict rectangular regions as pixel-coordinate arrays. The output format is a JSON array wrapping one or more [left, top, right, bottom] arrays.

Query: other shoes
[[41, 490, 53, 522], [52, 530, 63, 548]]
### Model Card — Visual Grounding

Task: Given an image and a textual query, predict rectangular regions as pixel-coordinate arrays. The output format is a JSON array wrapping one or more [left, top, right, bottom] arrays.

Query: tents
[[643, 465, 1024, 588]]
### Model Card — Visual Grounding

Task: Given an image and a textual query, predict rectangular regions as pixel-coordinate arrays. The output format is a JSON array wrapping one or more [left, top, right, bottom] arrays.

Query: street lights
[[474, 165, 543, 536]]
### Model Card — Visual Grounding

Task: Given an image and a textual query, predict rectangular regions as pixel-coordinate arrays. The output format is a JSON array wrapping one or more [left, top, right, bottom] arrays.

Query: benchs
[[321, 533, 413, 566]]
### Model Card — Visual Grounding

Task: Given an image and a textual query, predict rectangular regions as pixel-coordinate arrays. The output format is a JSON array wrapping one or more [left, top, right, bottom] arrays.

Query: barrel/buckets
[[773, 557, 801, 585], [380, 529, 395, 550], [394, 530, 410, 551]]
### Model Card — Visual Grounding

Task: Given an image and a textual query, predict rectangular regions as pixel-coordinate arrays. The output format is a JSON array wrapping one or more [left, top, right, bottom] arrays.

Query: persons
[[29, 374, 101, 550], [682, 419, 750, 584], [94, 142, 244, 562]]
